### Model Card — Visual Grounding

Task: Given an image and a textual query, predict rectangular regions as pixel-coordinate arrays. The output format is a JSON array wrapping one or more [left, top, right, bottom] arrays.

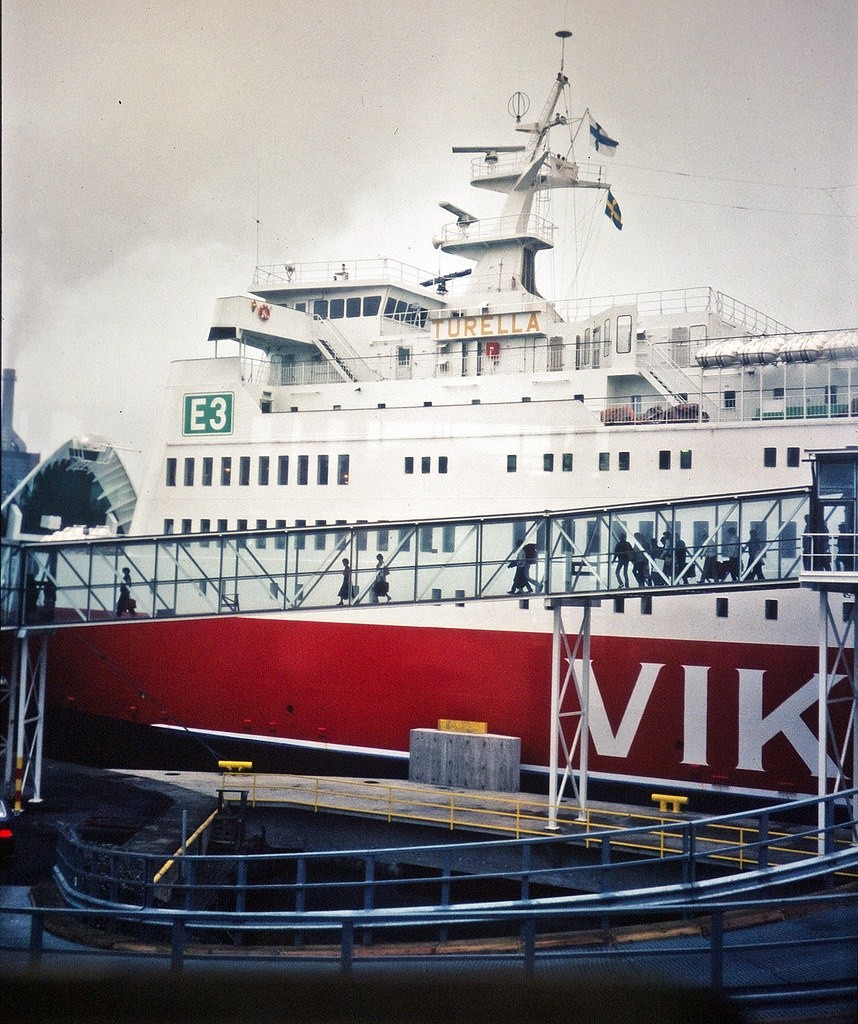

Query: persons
[[506, 540, 534, 596], [801, 513, 855, 571], [25, 573, 44, 625], [610, 527, 773, 588], [372, 554, 392, 604], [336, 558, 360, 606], [513, 540, 543, 594], [113, 567, 137, 621], [43, 576, 58, 625]]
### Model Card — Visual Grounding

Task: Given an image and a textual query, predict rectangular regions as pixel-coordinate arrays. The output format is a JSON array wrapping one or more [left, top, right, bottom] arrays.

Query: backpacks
[[524, 543, 536, 564]]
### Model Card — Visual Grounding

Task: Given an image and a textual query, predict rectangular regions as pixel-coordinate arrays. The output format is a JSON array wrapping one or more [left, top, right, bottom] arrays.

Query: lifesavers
[[257, 305, 270, 320]]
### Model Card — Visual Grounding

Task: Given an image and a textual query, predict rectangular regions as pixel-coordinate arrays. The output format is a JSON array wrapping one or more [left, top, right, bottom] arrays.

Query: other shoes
[[528, 589, 533, 593], [507, 590, 515, 594], [697, 580, 705, 583], [538, 585, 543, 593], [337, 602, 344, 605], [618, 584, 624, 589], [516, 590, 524, 594], [387, 596, 391, 603], [626, 586, 630, 590], [638, 583, 645, 588]]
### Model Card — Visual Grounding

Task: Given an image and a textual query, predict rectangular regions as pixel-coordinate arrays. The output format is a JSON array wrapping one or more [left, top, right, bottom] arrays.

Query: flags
[[589, 116, 619, 158], [605, 190, 623, 231]]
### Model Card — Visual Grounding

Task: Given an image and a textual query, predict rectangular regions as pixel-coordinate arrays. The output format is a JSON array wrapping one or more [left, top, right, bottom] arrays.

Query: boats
[[0, 29, 858, 825]]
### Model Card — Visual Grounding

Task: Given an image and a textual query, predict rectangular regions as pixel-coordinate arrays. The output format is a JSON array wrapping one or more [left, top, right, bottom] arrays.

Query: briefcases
[[706, 562, 725, 579]]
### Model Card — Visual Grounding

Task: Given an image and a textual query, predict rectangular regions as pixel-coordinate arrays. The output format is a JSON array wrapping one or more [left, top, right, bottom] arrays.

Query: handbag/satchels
[[129, 599, 136, 608], [724, 561, 744, 572], [627, 548, 635, 561], [374, 582, 389, 592], [653, 559, 665, 573], [385, 569, 390, 575], [682, 562, 696, 577]]
